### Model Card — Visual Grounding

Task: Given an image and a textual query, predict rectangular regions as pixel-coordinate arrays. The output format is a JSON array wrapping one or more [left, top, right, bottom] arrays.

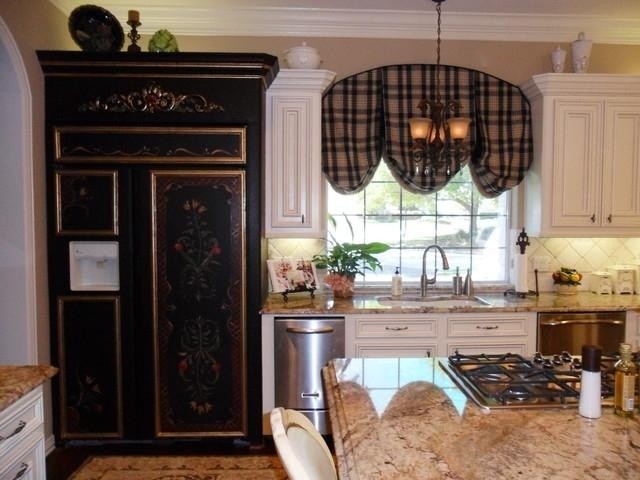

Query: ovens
[[537, 311, 628, 355]]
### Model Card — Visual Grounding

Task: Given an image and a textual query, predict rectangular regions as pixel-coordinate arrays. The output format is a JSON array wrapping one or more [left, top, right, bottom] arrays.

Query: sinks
[[373, 295, 494, 307]]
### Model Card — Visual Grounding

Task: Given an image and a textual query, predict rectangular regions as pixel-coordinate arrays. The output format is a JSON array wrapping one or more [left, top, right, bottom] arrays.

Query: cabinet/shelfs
[[345, 313, 536, 359], [518, 73, 640, 240], [0, 384, 47, 480], [261, 69, 337, 238], [34, 46, 280, 455]]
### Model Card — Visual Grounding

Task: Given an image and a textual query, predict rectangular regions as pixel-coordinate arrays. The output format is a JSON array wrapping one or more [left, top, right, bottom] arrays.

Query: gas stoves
[[449, 352, 640, 405]]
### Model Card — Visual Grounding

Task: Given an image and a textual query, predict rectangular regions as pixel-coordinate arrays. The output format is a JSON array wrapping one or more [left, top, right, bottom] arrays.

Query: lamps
[[405, 0, 473, 175]]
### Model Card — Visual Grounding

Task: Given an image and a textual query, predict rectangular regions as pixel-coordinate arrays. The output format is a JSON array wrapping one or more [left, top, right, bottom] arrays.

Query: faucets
[[420, 244, 449, 296]]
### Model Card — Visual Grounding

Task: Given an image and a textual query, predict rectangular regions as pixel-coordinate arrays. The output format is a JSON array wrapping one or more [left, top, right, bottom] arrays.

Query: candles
[[128, 9, 139, 22]]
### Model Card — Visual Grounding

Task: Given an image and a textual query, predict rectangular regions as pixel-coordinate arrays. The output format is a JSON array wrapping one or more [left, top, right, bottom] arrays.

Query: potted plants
[[310, 216, 394, 298]]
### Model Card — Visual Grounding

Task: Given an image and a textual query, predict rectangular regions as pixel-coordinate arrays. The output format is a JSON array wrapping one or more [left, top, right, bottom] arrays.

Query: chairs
[[269, 405, 338, 480]]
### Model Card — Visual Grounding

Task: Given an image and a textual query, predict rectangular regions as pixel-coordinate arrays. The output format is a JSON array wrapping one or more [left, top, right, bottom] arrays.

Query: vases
[[570, 32, 592, 73], [551, 44, 568, 73]]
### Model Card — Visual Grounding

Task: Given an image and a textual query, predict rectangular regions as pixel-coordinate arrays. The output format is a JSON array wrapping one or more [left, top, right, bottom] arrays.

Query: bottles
[[452, 266, 462, 295], [613, 341, 638, 419], [580, 350, 604, 421], [463, 268, 475, 296]]
